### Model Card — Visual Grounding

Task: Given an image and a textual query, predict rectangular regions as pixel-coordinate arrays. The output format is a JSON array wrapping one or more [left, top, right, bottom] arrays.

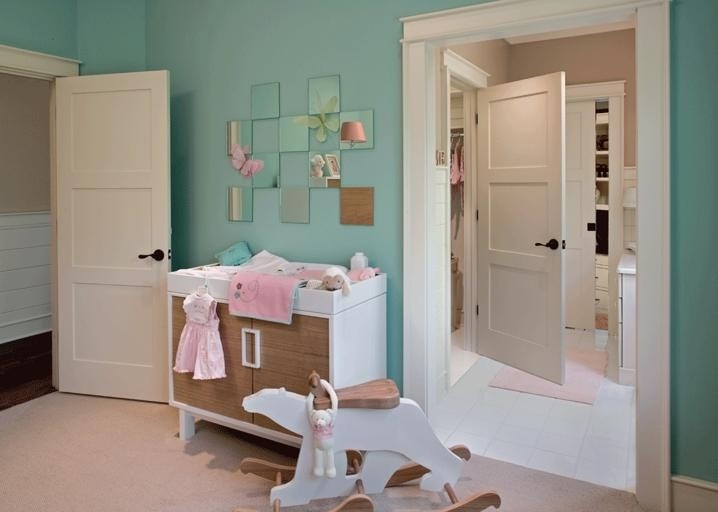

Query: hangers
[[183, 279, 218, 309]]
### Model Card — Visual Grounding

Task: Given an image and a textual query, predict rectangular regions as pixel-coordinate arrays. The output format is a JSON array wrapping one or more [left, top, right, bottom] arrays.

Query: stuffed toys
[[305, 266, 353, 299]]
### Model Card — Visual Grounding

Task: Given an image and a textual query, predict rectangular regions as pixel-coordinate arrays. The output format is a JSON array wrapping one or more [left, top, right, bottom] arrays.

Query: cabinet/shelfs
[[166, 262, 388, 449], [593, 101, 609, 328]]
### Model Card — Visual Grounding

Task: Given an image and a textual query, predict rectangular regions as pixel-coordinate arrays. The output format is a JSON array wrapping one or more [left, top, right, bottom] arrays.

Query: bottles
[[350, 252, 368, 272]]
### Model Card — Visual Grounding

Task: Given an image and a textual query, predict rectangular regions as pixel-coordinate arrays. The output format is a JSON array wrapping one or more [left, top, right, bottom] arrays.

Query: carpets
[[487, 345, 609, 406], [1, 385, 640, 511]]
